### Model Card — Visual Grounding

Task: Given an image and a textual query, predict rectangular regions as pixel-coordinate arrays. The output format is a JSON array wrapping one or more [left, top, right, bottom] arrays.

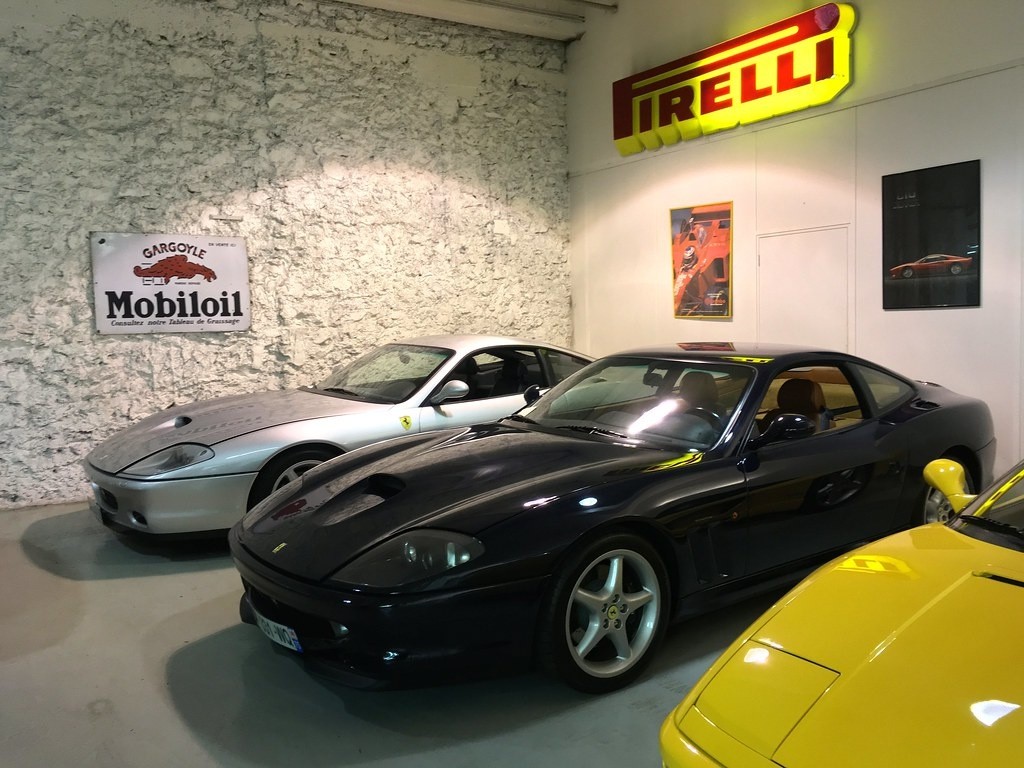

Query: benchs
[[482, 369, 562, 389], [726, 378, 900, 435]]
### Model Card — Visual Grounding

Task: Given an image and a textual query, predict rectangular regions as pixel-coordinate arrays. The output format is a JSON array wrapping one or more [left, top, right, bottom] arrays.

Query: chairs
[[677, 371, 725, 429], [490, 357, 530, 396], [441, 358, 479, 403], [758, 379, 837, 440]]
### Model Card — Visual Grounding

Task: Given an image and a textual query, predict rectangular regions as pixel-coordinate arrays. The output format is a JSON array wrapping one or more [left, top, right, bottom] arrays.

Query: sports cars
[[228, 343, 997, 699], [80, 337, 610, 539]]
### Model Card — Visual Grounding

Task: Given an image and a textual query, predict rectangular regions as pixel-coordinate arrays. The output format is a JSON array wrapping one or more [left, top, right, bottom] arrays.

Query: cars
[[889, 253, 972, 280], [660, 457, 1024, 767]]
